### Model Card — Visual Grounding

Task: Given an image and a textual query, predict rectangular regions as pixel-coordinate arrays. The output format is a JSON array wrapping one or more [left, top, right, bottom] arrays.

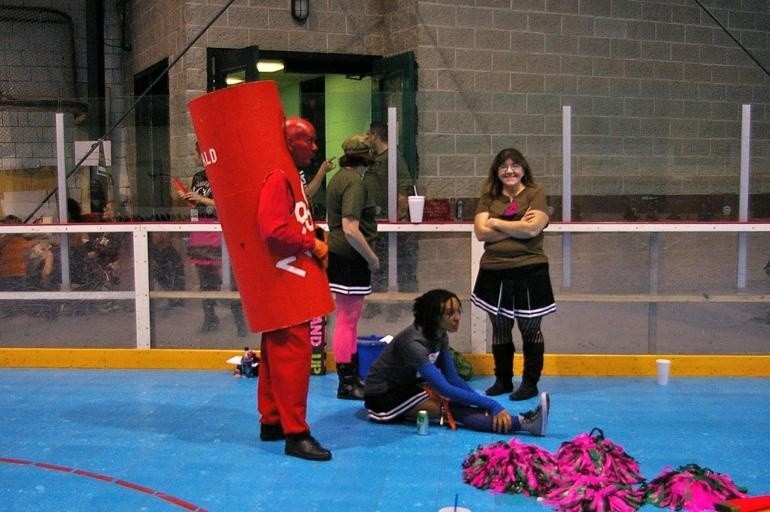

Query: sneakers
[[518, 392, 550, 437]]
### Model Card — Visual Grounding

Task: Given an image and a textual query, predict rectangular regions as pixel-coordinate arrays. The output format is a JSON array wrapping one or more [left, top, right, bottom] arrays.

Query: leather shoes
[[259, 423, 285, 441], [286, 432, 331, 461]]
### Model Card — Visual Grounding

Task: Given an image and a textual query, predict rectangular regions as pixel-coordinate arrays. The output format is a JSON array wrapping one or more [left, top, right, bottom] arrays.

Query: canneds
[[416, 409, 429, 436]]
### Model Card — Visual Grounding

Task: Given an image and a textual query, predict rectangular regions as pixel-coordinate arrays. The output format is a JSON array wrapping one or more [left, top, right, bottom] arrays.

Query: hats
[[342, 134, 371, 155]]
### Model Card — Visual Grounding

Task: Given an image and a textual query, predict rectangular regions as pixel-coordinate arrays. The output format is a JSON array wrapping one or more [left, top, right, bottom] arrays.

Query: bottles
[[241, 346, 252, 382]]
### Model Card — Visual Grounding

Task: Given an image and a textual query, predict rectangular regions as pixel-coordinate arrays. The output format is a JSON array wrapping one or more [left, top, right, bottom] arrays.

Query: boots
[[336, 351, 367, 400], [509, 340, 544, 401], [486, 342, 514, 396]]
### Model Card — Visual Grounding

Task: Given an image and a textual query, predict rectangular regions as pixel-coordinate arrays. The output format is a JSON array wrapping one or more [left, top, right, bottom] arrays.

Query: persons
[[465, 147, 558, 401], [358, 119, 420, 324], [325, 135, 383, 401], [175, 139, 251, 339], [251, 114, 333, 462], [0, 151, 189, 325], [362, 285, 549, 437]]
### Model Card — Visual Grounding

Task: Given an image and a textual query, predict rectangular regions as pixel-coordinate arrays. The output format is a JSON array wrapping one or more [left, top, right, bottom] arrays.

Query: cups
[[408, 196, 426, 223], [656, 358, 671, 386]]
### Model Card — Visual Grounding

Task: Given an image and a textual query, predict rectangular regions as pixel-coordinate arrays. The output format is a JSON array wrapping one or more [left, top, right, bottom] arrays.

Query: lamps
[[291, 0, 309, 25]]
